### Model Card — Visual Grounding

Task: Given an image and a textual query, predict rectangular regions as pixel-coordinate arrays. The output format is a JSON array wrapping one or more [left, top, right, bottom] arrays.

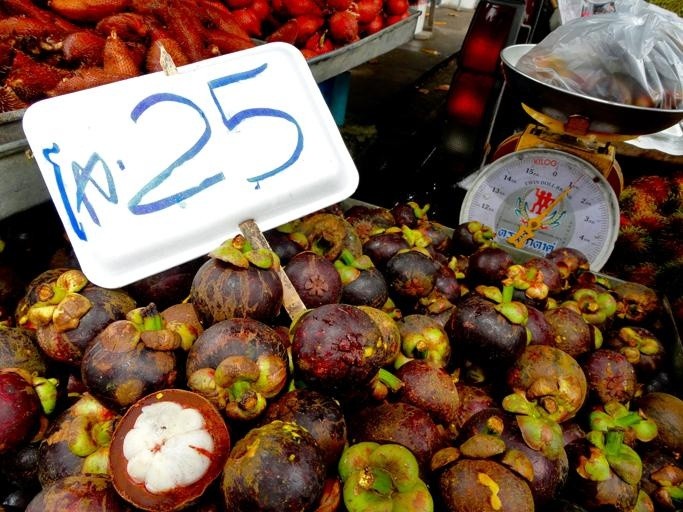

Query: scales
[[456, 44, 683, 272]]
